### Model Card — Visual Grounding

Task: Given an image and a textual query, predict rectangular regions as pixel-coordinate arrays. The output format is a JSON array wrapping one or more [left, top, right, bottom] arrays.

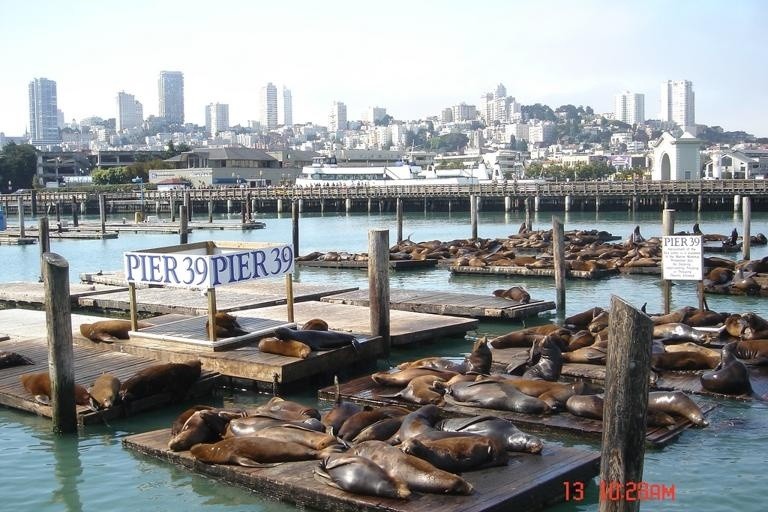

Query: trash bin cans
[[0, 210, 6, 231]]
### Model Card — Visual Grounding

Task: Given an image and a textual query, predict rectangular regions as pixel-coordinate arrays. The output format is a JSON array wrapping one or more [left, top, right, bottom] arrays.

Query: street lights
[[132, 176, 144, 211]]
